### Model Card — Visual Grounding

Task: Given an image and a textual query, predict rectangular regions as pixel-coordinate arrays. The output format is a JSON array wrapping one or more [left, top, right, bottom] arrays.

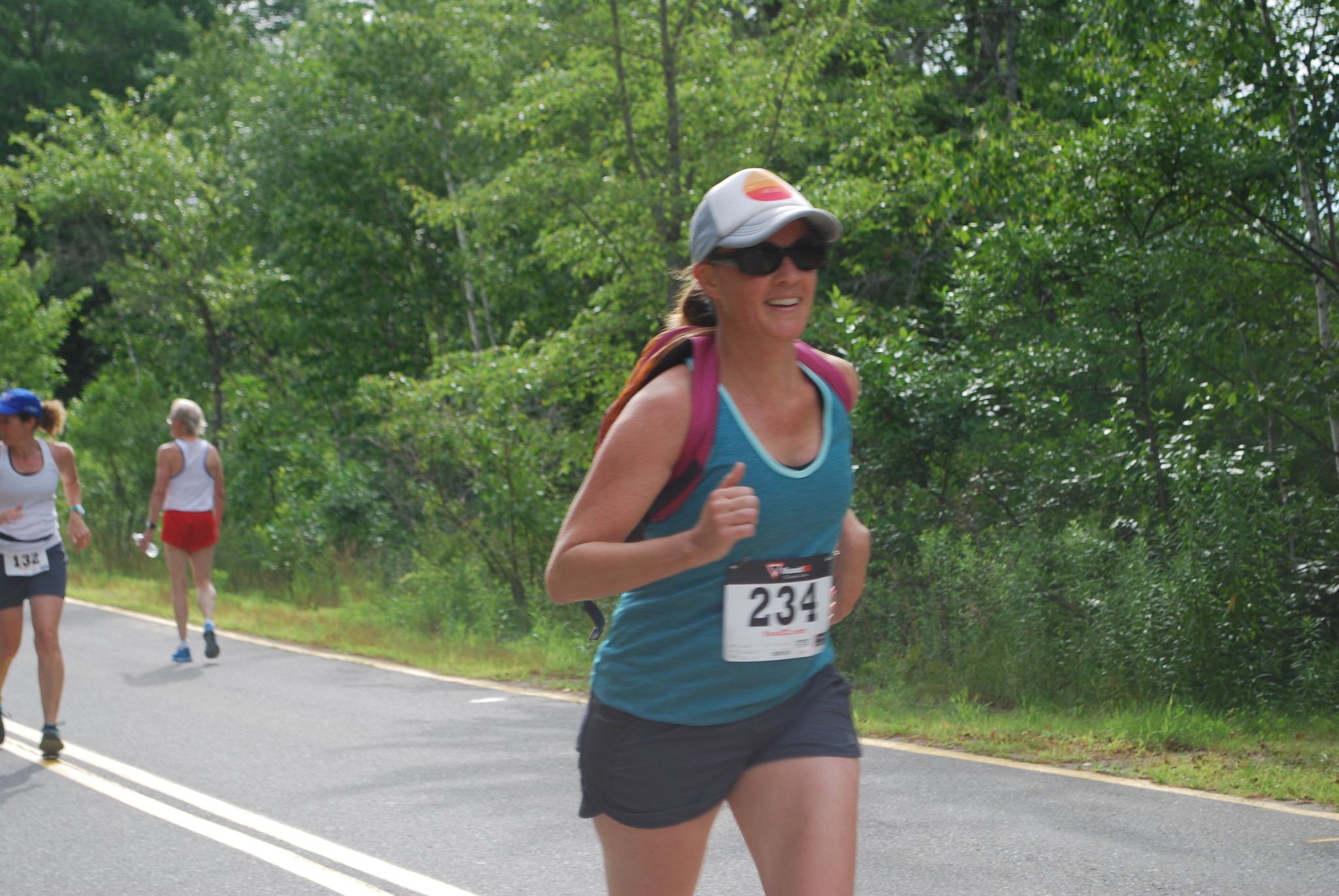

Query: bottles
[[133, 533, 159, 558]]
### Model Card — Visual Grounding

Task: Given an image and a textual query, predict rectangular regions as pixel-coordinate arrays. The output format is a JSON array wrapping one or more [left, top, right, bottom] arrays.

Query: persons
[[139, 397, 224, 665], [0, 389, 92, 763], [543, 169, 870, 895]]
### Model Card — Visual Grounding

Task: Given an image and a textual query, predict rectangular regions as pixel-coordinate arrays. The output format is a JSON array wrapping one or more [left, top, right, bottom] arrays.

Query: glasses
[[705, 236, 830, 277]]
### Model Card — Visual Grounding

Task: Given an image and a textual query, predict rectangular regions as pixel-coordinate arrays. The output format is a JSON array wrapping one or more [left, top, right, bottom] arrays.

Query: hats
[[2, 389, 41, 416], [691, 168, 843, 266]]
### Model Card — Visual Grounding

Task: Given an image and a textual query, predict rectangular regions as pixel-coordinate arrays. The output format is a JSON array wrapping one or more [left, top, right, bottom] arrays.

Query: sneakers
[[204, 629, 222, 658], [0, 707, 5, 743], [173, 648, 189, 660], [40, 724, 63, 760]]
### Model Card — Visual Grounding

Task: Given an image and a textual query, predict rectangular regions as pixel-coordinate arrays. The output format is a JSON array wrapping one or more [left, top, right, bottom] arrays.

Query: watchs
[[68, 504, 86, 517], [145, 521, 157, 530]]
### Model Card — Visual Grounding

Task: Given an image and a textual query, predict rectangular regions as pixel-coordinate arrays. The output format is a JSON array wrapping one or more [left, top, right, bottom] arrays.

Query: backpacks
[[595, 325, 859, 545]]
